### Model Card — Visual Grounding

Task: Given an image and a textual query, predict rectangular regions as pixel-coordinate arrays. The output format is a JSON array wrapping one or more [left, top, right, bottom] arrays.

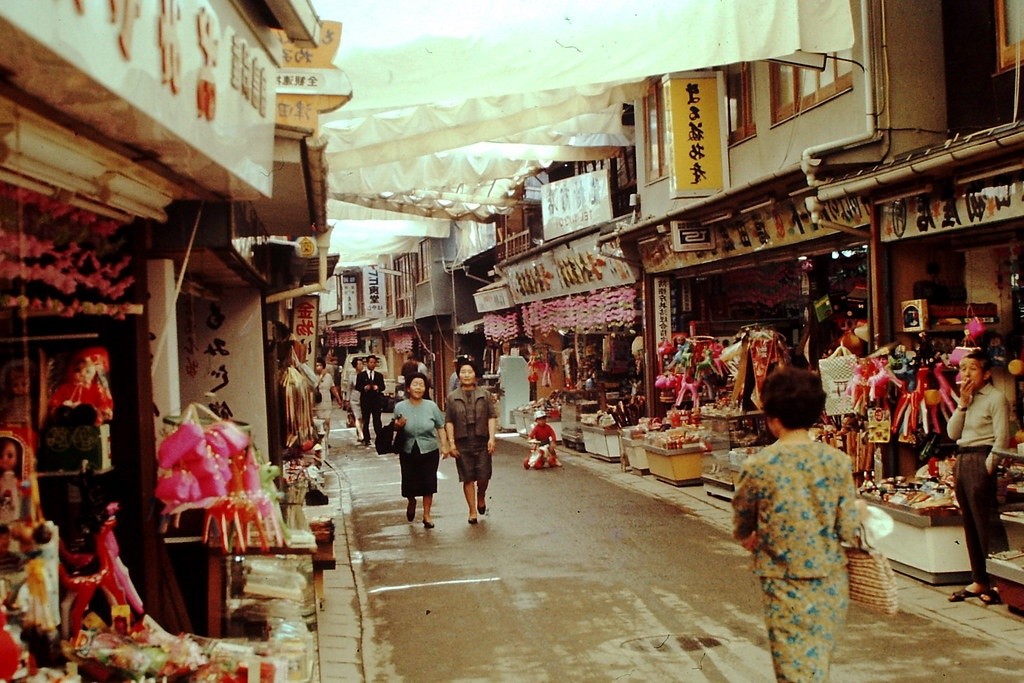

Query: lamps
[[379, 268, 406, 277], [760, 47, 866, 74]]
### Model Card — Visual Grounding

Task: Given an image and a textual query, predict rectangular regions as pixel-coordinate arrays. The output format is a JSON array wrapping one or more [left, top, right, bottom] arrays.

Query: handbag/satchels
[[844, 522, 898, 617], [346, 407, 356, 428], [156, 401, 261, 506], [818, 346, 860, 416]]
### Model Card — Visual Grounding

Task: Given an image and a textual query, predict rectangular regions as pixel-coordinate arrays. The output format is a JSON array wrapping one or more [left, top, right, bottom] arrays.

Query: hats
[[534, 411, 551, 422]]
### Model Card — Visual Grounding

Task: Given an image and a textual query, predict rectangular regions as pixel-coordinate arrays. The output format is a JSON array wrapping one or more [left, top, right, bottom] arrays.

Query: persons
[[311, 343, 387, 454], [376, 371, 451, 529], [398, 348, 432, 399], [524, 410, 562, 469], [732, 365, 899, 682], [445, 360, 498, 524], [946, 347, 1012, 608], [0, 344, 154, 669]]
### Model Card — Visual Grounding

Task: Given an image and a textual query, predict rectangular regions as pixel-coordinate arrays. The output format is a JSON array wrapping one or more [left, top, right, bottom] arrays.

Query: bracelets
[[957, 404, 968, 412]]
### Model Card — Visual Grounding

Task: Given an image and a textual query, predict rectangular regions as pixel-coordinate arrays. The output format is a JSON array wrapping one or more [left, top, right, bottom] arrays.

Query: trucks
[[339, 353, 395, 413]]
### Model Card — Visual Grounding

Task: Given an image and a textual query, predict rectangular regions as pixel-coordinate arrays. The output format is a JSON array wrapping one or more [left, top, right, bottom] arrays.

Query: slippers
[[949, 586, 985, 601], [979, 588, 1003, 605]]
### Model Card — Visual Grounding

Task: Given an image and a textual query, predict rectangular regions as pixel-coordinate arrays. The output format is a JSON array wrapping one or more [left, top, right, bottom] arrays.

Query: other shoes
[[365, 440, 371, 445], [468, 518, 477, 524], [423, 520, 434, 528], [406, 499, 416, 522], [477, 504, 486, 514]]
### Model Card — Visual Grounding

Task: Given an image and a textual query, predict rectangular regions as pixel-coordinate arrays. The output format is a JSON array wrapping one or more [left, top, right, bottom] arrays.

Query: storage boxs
[[900, 298, 930, 332]]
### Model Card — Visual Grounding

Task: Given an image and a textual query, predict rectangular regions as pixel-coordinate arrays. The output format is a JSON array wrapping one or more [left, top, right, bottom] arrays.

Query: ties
[[370, 370, 372, 383]]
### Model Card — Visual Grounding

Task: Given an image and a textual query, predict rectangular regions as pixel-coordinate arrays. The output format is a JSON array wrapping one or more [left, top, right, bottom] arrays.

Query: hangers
[[740, 322, 787, 344]]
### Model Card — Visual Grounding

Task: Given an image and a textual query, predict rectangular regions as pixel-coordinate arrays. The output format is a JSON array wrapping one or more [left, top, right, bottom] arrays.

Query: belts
[[957, 445, 993, 453]]
